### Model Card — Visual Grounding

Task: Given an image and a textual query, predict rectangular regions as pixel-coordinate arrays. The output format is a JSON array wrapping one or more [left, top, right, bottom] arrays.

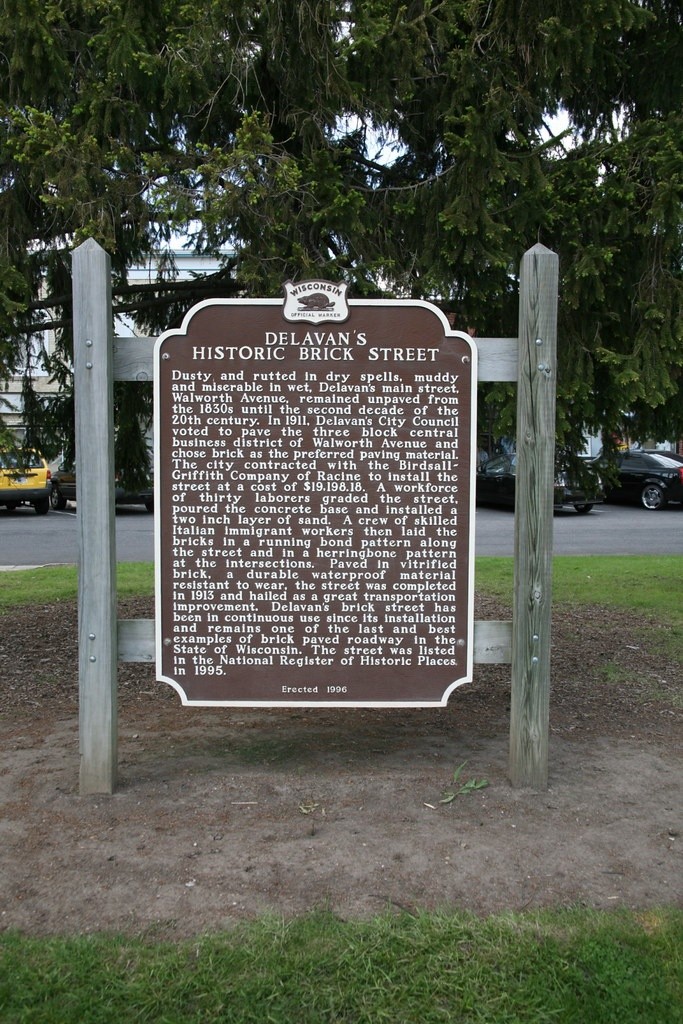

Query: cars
[[474, 451, 605, 515], [589, 449, 683, 511], [50, 448, 155, 513]]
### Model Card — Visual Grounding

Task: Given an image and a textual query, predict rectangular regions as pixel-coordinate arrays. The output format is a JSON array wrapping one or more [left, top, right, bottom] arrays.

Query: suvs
[[0, 447, 52, 515]]
